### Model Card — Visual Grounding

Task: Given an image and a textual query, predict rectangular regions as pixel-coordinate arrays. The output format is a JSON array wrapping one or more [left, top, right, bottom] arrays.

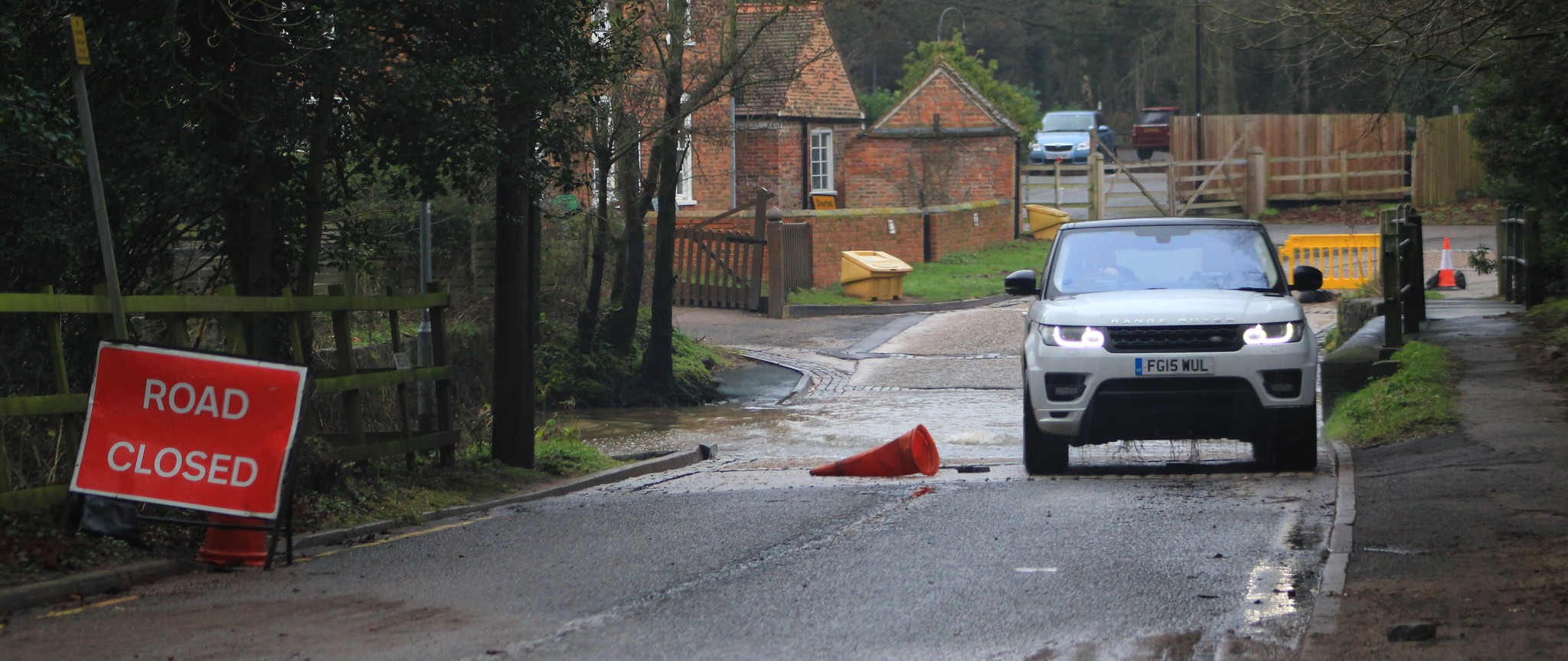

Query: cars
[[1025, 110, 1118, 175]]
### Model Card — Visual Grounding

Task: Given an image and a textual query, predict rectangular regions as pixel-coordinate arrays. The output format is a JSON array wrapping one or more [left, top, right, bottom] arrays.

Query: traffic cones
[[176, 513, 271, 571], [808, 425, 940, 479], [1432, 236, 1465, 290]]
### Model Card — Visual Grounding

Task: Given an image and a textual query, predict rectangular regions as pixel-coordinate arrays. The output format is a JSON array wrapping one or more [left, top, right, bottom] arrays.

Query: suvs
[[1130, 107, 1188, 160], [1004, 216, 1324, 476]]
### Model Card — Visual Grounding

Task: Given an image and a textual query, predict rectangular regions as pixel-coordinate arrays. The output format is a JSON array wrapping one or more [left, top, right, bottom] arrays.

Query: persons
[[1070, 236, 1140, 287]]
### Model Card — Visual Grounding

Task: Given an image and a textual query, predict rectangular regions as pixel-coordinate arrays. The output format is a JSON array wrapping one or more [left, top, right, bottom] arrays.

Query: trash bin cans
[[1025, 204, 1070, 240], [840, 250, 913, 302]]
